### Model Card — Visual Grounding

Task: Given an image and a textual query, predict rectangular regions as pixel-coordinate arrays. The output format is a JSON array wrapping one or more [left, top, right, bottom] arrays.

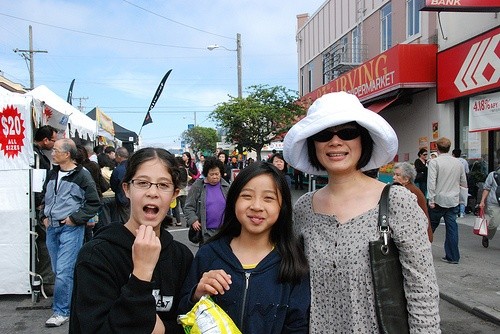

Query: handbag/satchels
[[188, 222, 202, 244], [473, 206, 488, 236], [369, 182, 411, 334]]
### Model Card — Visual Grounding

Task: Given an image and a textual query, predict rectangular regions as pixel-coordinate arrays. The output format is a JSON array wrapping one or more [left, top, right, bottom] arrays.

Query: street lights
[[208, 32, 244, 100]]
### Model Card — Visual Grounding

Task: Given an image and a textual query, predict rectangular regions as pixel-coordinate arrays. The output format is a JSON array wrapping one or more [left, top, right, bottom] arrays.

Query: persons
[[479, 166, 500, 248], [282, 92, 441, 334], [414, 148, 469, 217], [426, 138, 468, 264], [177, 162, 311, 334], [164, 151, 254, 226], [37, 138, 101, 327], [33, 126, 131, 243], [184, 157, 231, 248], [392, 162, 432, 243], [264, 149, 303, 192], [68, 147, 194, 334]]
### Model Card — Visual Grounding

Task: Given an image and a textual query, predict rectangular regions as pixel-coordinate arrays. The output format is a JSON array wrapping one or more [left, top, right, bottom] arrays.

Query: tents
[[86, 107, 137, 154], [0, 86, 98, 304]]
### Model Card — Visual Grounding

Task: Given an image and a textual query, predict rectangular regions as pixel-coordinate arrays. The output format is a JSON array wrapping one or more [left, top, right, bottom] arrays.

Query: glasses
[[423, 154, 427, 156], [313, 127, 360, 142], [127, 179, 174, 191]]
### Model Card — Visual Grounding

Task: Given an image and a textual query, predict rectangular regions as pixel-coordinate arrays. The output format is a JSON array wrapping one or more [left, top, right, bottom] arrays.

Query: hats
[[282, 90, 399, 176]]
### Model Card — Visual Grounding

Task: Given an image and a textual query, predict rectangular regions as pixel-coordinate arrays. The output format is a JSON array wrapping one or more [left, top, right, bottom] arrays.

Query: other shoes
[[482, 236, 489, 248], [175, 223, 182, 226], [440, 256, 459, 264]]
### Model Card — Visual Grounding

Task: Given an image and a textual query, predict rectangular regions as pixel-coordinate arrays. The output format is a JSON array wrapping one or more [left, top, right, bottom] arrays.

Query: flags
[[67, 79, 75, 105], [142, 69, 172, 127]]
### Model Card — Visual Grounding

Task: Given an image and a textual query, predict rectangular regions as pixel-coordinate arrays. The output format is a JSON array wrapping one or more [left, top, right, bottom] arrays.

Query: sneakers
[[46, 313, 69, 327]]
